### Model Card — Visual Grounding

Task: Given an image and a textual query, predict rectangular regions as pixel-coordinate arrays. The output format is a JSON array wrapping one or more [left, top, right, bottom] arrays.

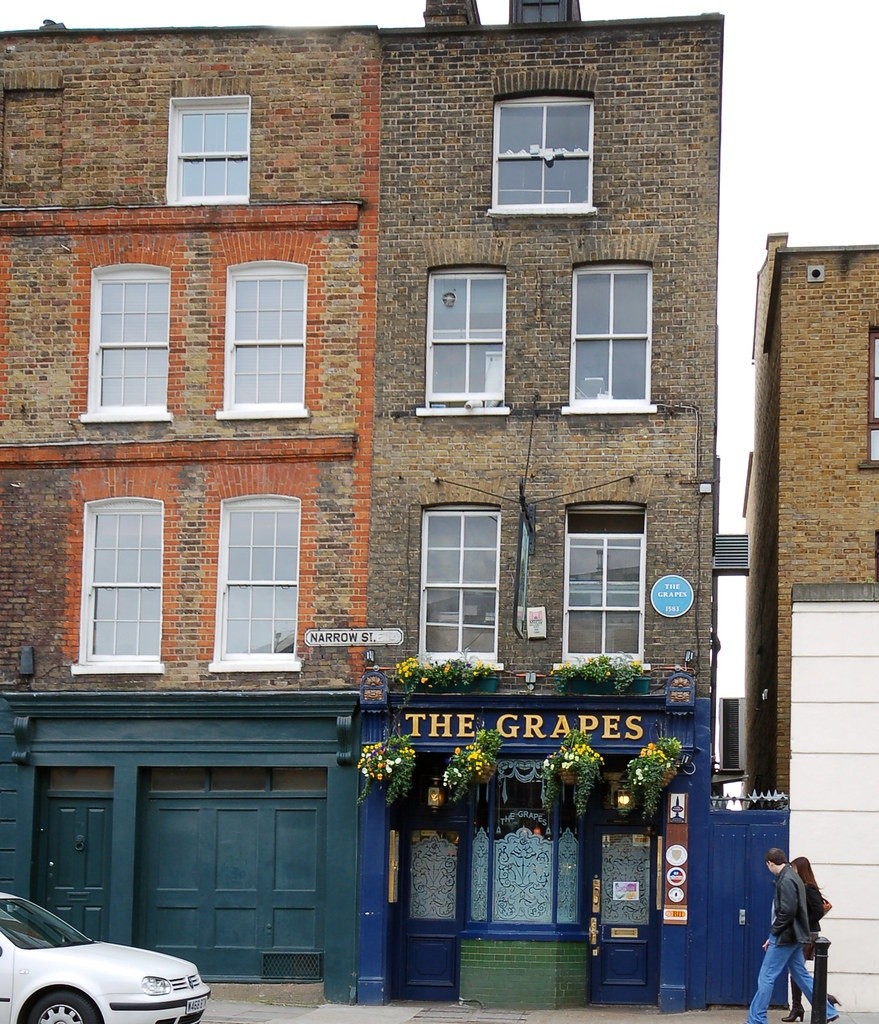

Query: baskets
[[469, 732, 497, 784], [644, 725, 677, 790], [554, 733, 581, 784]]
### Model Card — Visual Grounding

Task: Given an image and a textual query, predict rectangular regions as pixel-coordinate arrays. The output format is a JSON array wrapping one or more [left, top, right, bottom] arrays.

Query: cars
[[0, 890, 212, 1024]]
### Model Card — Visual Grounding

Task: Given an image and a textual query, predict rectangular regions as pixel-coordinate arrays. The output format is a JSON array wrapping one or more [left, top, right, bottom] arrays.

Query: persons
[[742, 848, 843, 1024]]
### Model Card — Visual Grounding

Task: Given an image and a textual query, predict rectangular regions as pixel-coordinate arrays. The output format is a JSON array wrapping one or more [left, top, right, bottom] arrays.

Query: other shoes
[[827, 1014, 840, 1024]]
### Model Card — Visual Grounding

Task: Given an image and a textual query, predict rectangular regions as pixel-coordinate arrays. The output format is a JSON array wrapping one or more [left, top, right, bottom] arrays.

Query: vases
[[404, 676, 497, 693], [562, 676, 650, 694]]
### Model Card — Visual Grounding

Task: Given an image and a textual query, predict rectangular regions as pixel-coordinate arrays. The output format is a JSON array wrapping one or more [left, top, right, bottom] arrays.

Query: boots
[[827, 994, 841, 1008], [782, 976, 804, 1022]]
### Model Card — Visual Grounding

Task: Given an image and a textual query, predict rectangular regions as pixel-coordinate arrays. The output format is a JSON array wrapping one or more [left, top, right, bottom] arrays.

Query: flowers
[[558, 657, 643, 693], [627, 736, 681, 818], [539, 729, 605, 818], [356, 735, 417, 807], [442, 729, 503, 801], [396, 657, 487, 689]]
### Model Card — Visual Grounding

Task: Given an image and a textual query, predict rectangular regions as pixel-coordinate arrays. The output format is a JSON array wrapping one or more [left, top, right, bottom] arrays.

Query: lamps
[[615, 780, 636, 817], [428, 777, 445, 815]]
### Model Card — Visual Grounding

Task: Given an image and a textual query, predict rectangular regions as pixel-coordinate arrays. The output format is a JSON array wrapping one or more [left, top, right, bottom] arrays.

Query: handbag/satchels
[[821, 897, 832, 917]]
